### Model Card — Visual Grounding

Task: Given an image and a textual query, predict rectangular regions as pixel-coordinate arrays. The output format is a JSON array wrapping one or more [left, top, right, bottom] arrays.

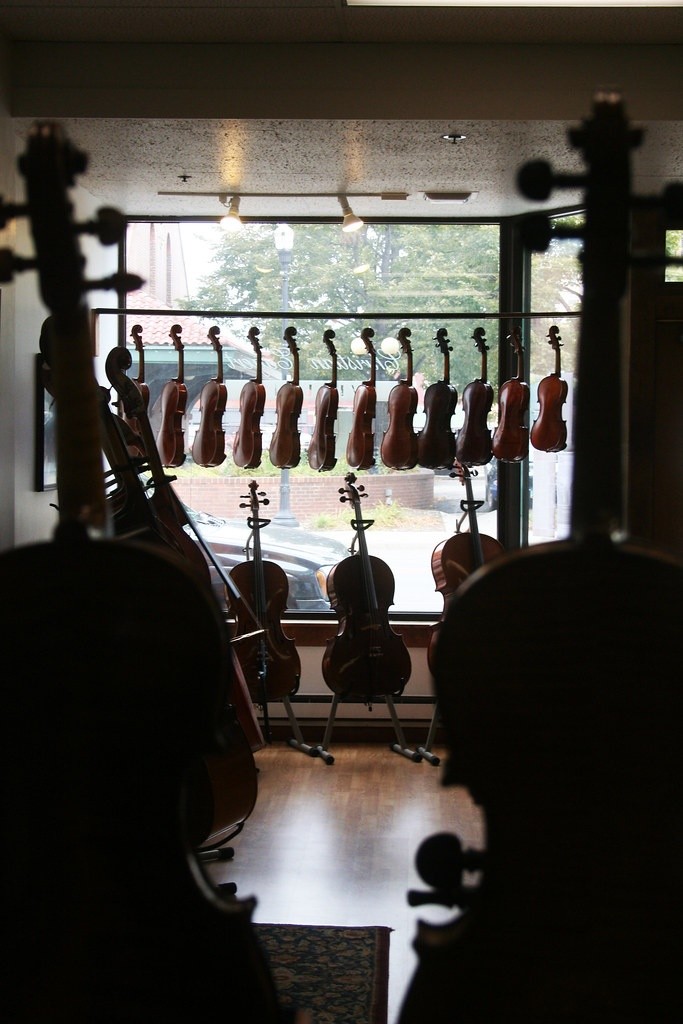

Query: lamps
[[337, 194, 365, 233], [218, 193, 246, 236]]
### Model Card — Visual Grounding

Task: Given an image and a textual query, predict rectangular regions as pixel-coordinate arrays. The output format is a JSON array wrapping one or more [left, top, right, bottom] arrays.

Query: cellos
[[427, 458, 504, 680], [97, 347, 267, 840], [227, 480, 302, 703], [321, 471, 412, 697]]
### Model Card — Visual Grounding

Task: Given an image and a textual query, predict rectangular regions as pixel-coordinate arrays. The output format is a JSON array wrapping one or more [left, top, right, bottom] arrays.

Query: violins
[[395, 86, 683, 1024], [0, 120, 282, 1024], [112, 324, 569, 473]]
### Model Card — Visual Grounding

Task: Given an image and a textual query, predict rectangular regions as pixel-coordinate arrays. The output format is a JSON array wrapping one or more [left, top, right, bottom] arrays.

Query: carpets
[[253, 922, 394, 1024]]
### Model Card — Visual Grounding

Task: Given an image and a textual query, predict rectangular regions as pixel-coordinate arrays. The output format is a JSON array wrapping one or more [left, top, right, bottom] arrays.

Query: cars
[[139, 472, 352, 613], [486, 467, 534, 511]]
